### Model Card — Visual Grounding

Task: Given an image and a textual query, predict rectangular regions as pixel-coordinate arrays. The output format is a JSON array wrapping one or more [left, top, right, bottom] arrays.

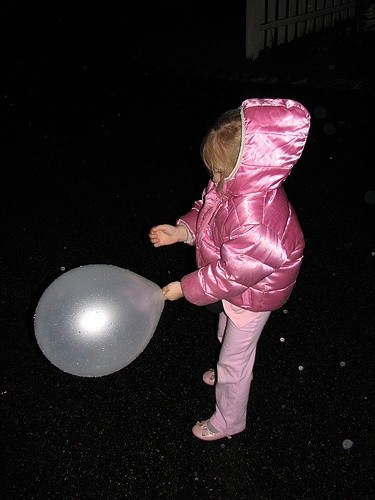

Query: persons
[[149, 98, 311, 440]]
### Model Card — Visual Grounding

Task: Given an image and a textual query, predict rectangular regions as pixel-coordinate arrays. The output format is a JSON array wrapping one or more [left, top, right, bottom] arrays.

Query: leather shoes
[[192, 419, 232, 441], [203, 368, 218, 386]]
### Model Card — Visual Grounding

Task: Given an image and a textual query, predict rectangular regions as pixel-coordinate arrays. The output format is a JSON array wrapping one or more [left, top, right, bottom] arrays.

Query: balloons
[[34, 265, 166, 379]]
